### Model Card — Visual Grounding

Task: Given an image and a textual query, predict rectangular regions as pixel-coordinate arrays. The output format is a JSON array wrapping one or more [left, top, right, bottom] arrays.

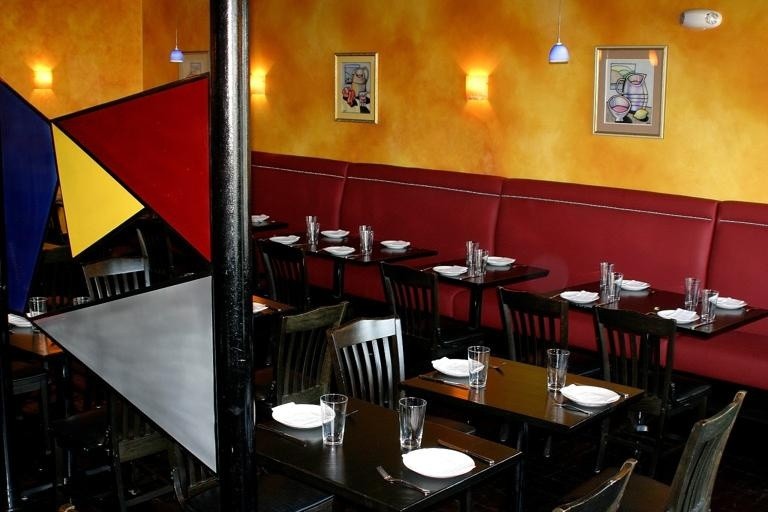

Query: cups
[[608, 272, 624, 302], [542, 393, 564, 425], [319, 393, 350, 446], [360, 255, 371, 263], [467, 387, 485, 405], [700, 288, 720, 322], [684, 306, 697, 313], [600, 261, 614, 288], [473, 248, 489, 276], [546, 347, 571, 392], [700, 322, 713, 334], [684, 277, 702, 306], [358, 224, 372, 235], [467, 345, 491, 388], [466, 240, 481, 267], [307, 221, 321, 241], [73, 296, 92, 308], [305, 215, 317, 237], [359, 230, 374, 256], [398, 397, 427, 450], [29, 296, 48, 333]]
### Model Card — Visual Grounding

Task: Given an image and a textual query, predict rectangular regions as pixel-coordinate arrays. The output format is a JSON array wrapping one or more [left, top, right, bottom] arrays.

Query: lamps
[[170, 25, 184, 64], [548, 1, 570, 63]]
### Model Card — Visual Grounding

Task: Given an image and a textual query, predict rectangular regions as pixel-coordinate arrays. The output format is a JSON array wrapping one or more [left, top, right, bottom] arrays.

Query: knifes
[[574, 382, 630, 399], [437, 437, 495, 466], [688, 319, 718, 331], [257, 423, 309, 448], [418, 374, 472, 391]]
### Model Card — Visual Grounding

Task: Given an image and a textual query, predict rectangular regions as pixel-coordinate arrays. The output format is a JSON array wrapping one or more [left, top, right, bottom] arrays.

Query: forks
[[488, 362, 508, 370], [548, 394, 592, 415], [376, 465, 431, 497]]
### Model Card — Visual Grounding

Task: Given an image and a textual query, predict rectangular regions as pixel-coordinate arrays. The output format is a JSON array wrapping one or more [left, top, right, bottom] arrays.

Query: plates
[[251, 222, 270, 227], [483, 265, 511, 272], [431, 356, 485, 378], [10, 327, 34, 335], [432, 265, 468, 276], [559, 290, 600, 304], [320, 231, 346, 239], [710, 297, 748, 310], [251, 215, 265, 222], [402, 446, 477, 480], [380, 248, 408, 254], [615, 280, 651, 291], [321, 237, 343, 243], [269, 236, 293, 244], [714, 308, 745, 316], [380, 240, 411, 249], [322, 245, 356, 256], [618, 289, 650, 297], [657, 309, 700, 325], [482, 256, 516, 267], [270, 401, 336, 430], [274, 421, 329, 445], [253, 302, 265, 314], [677, 320, 698, 329], [15, 316, 31, 328], [432, 372, 476, 384], [559, 384, 619, 407]]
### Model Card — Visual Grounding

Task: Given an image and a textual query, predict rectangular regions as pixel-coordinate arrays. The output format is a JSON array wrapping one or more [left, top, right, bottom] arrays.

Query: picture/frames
[[595, 47, 667, 140], [334, 53, 378, 125], [177, 53, 209, 81]]
